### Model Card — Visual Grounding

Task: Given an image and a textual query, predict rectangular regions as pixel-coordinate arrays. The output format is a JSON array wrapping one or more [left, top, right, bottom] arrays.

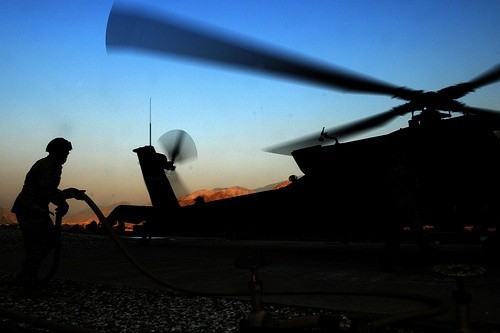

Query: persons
[[10, 138, 72, 299]]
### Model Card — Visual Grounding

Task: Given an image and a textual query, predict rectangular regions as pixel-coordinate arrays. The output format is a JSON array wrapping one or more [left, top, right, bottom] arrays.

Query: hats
[[45, 139, 71, 151]]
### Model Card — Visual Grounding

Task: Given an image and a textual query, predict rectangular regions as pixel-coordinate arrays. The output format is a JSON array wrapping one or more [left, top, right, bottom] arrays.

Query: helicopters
[[99, 4, 500, 275]]
